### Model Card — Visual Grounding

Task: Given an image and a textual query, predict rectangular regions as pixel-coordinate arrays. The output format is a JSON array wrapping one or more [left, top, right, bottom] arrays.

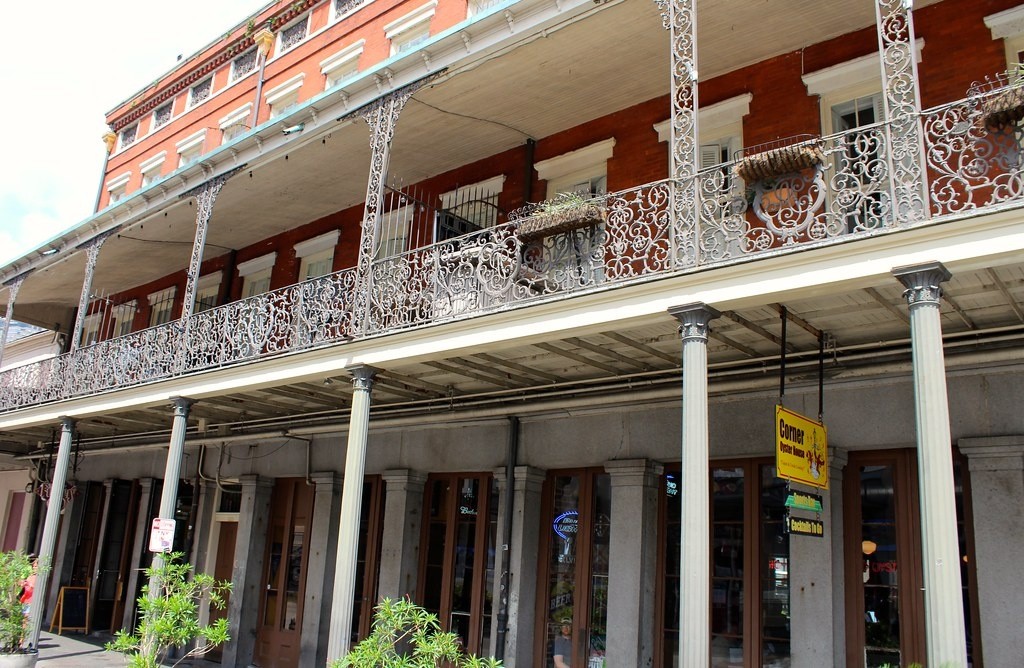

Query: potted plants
[[743, 178, 798, 212], [512, 192, 604, 243], [0, 549, 53, 668]]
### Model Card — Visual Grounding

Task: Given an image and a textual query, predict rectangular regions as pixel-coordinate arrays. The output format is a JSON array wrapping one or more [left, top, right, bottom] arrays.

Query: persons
[[763, 599, 790, 661], [711, 623, 743, 668], [8, 554, 39, 653], [554, 618, 572, 668]]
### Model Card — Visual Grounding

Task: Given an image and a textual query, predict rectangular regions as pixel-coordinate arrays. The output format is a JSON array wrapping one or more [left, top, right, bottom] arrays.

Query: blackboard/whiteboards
[[60, 587, 90, 630]]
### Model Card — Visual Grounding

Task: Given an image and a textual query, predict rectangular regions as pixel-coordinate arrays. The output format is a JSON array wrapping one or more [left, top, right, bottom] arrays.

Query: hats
[[561, 616, 573, 624]]
